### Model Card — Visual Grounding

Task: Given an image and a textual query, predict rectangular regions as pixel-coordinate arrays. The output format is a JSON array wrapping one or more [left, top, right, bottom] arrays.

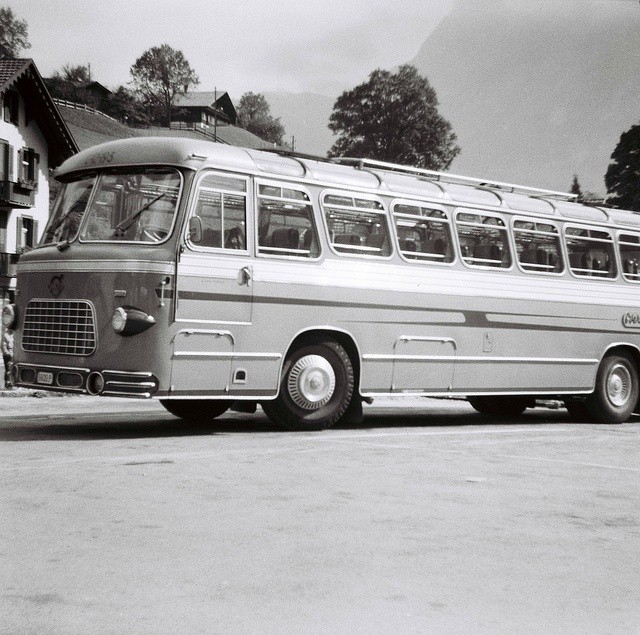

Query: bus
[[1, 136, 640, 431]]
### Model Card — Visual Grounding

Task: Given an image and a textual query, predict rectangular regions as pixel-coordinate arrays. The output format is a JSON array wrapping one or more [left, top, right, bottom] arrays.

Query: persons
[[2, 328, 14, 388]]
